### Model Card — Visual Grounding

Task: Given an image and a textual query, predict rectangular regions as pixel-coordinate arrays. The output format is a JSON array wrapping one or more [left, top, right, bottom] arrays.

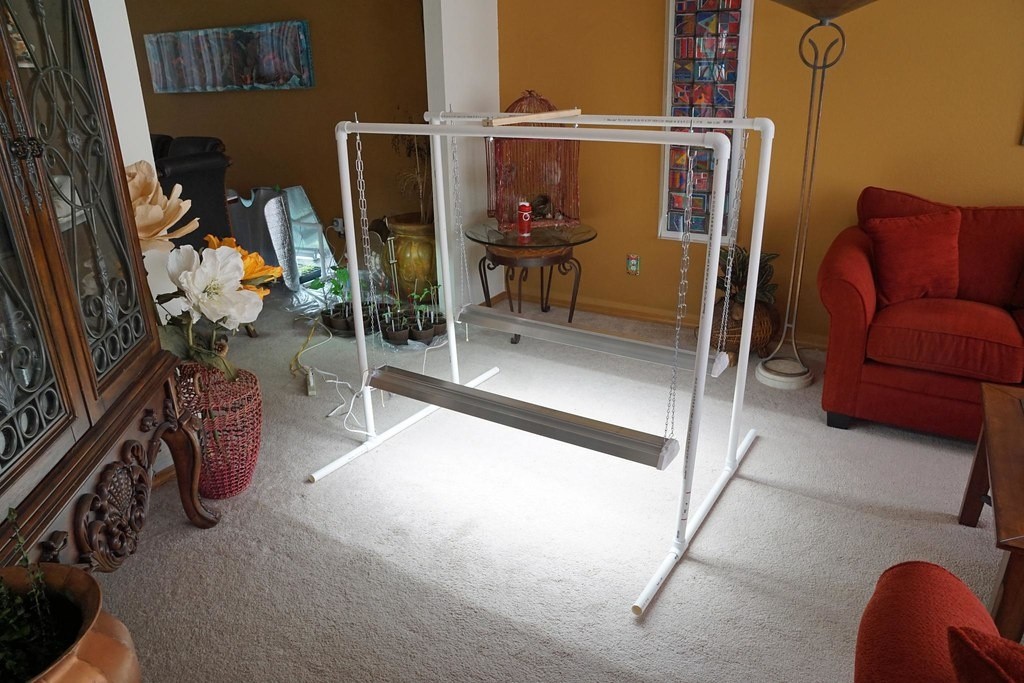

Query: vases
[[175, 364, 261, 500]]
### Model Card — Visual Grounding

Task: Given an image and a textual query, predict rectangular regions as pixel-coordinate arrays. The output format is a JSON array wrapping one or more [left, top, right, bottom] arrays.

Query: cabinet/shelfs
[[0, 0, 224, 574]]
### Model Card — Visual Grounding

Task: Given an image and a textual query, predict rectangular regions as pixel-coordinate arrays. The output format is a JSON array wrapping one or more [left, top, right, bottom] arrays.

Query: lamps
[[363, 361, 680, 471], [456, 302, 729, 378]]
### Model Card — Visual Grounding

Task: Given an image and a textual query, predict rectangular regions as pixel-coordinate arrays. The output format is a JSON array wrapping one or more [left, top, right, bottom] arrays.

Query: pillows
[[865, 205, 961, 310], [946, 624, 1024, 683]]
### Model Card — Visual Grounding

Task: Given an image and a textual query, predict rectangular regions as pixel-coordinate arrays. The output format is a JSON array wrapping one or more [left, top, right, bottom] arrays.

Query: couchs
[[851, 561, 1000, 683], [150, 133, 233, 263], [815, 186, 1024, 442]]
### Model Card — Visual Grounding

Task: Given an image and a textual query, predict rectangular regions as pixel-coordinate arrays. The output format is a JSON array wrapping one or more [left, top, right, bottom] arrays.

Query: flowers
[[124, 158, 284, 385]]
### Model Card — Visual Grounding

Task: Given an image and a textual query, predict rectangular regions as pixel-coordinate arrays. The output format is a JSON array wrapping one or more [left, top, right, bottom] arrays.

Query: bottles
[[518, 202, 531, 236]]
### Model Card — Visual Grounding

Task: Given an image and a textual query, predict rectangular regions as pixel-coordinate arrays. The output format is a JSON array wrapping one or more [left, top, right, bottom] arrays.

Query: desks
[[463, 219, 598, 343]]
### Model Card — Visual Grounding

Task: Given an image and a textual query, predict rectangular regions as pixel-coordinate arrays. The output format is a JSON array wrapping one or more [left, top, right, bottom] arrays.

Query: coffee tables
[[958, 381, 1024, 644]]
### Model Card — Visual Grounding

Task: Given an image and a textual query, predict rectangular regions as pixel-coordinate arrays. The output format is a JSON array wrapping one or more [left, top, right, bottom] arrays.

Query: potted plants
[[708, 243, 782, 366], [0, 508, 141, 683], [300, 110, 447, 347]]
